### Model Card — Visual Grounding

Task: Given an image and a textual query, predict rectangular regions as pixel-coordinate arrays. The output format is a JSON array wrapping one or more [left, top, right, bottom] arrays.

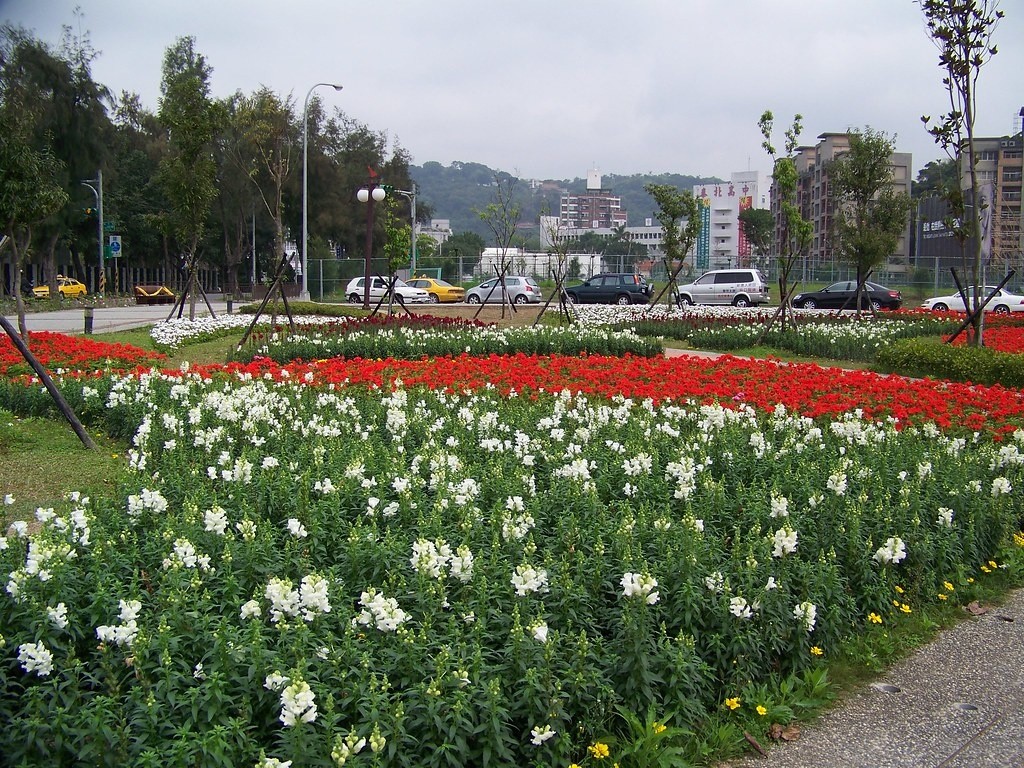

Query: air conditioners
[[1009, 141, 1015, 146], [1001, 141, 1007, 147]]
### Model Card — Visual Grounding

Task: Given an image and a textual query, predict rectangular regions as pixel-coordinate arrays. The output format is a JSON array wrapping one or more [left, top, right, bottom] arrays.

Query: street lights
[[300, 82, 344, 302], [358, 179, 386, 309]]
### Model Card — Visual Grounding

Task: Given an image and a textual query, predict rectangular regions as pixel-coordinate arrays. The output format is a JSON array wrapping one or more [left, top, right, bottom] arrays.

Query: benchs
[[134, 282, 176, 306]]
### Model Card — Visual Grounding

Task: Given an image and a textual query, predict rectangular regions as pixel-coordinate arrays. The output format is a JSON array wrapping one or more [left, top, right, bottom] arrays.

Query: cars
[[32, 275, 88, 301], [345, 277, 430, 305], [465, 277, 542, 305], [402, 278, 465, 304], [666, 268, 771, 307], [922, 286, 1024, 317], [788, 282, 903, 311]]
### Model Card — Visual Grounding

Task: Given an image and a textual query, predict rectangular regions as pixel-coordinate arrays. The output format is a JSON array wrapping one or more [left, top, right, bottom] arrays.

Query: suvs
[[560, 274, 655, 305]]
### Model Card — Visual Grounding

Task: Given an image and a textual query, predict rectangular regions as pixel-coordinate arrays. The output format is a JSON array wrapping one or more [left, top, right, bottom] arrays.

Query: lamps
[[84, 306, 94, 317], [226, 294, 234, 301]]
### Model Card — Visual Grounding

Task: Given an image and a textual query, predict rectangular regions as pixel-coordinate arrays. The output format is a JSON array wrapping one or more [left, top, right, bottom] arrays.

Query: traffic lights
[[379, 184, 394, 192], [82, 208, 98, 215]]
